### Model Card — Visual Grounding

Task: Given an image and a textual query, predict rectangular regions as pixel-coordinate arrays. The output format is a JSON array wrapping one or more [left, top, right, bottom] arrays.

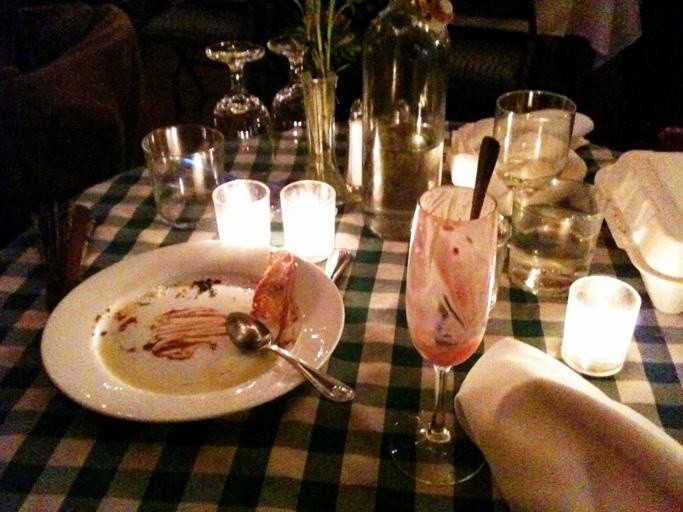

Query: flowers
[[289, 1, 459, 181]]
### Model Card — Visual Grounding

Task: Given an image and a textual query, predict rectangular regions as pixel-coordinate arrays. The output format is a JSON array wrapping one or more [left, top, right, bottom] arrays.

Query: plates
[[39, 240, 345, 423]]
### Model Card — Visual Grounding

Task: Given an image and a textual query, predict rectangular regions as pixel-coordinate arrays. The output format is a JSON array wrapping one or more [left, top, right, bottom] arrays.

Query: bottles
[[358, 0, 449, 242]]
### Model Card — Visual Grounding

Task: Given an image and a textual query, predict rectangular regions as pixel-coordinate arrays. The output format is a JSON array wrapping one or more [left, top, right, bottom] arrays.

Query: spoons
[[225, 312, 354, 402]]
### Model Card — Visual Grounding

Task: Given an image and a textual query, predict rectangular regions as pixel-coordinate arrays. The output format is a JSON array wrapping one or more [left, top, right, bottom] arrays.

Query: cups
[[140, 123, 224, 230], [507, 175, 606, 299], [211, 179, 270, 246], [279, 179, 336, 263], [561, 275, 641, 377], [492, 90, 576, 192]]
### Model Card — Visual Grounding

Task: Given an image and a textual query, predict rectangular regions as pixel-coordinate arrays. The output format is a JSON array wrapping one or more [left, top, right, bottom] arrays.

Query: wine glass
[[389, 184, 500, 488], [204, 38, 274, 173], [266, 31, 312, 170]]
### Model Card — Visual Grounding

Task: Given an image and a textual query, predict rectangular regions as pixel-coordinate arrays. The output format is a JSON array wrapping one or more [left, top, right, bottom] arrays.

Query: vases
[[295, 71, 362, 216]]
